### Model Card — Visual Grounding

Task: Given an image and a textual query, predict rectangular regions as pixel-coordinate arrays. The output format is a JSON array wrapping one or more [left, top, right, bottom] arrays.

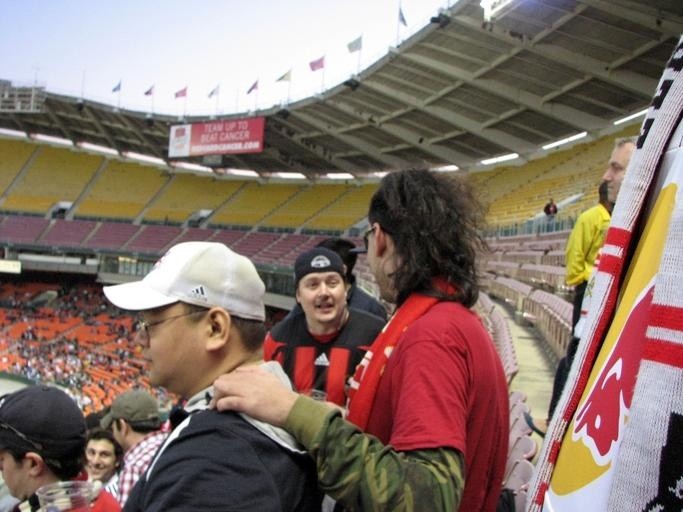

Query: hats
[[102, 242, 266, 323], [294, 249, 345, 281], [99, 390, 158, 431], [349, 247, 366, 252], [1, 385, 88, 457]]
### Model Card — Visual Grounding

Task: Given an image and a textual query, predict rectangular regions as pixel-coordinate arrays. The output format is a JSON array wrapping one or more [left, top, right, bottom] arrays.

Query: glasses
[[135, 310, 209, 329], [363, 227, 375, 245], [0, 394, 43, 450]]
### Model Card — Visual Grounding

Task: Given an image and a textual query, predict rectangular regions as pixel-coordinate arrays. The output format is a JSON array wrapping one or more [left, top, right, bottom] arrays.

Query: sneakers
[[524, 411, 545, 438]]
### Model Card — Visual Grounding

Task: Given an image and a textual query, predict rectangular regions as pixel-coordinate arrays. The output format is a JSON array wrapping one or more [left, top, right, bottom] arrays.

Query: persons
[[601, 135, 640, 205], [523, 32, 683, 512], [544, 180, 617, 427], [1, 281, 292, 511], [543, 197, 558, 222], [311, 235, 389, 324], [262, 246, 384, 408], [102, 241, 322, 512], [206, 167, 510, 511]]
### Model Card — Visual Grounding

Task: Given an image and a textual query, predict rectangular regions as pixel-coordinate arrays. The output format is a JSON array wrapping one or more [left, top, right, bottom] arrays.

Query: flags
[[398, 7, 408, 28], [347, 36, 363, 53], [309, 56, 326, 71], [174, 87, 188, 98], [208, 84, 220, 98], [277, 71, 291, 81], [245, 81, 259, 95], [144, 87, 153, 95], [112, 84, 122, 92]]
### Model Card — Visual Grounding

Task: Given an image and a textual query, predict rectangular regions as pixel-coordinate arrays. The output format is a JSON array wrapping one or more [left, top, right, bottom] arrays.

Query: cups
[[34, 480, 92, 512]]
